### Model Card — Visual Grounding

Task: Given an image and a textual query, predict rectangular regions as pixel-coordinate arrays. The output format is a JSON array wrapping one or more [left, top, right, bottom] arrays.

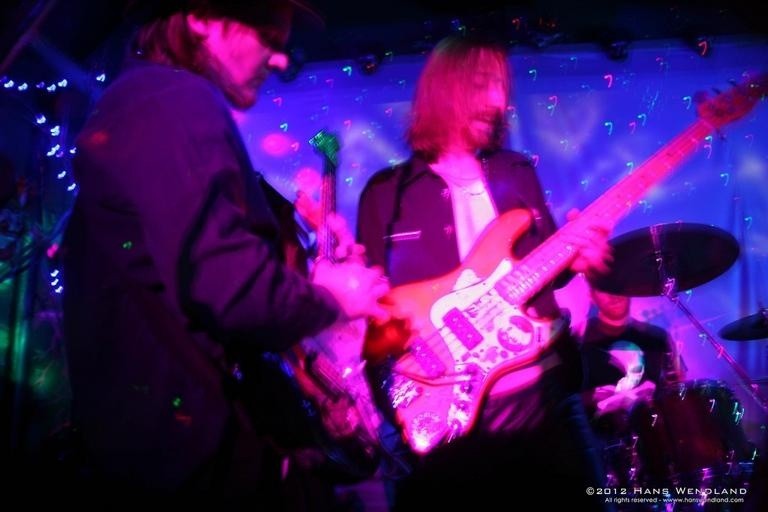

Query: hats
[[276, 2, 330, 30]]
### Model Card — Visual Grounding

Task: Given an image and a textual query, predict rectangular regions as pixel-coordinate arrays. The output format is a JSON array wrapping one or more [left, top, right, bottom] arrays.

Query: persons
[[63, 0, 391, 512], [582, 289, 680, 439], [357, 31, 615, 511]]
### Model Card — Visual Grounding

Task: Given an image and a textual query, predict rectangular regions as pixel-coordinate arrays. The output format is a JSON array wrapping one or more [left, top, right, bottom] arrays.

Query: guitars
[[290, 132, 369, 435], [364, 69, 762, 456]]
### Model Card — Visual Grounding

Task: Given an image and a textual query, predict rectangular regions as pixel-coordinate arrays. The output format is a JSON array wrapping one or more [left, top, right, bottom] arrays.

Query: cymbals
[[717, 308, 767, 341], [584, 222, 740, 297]]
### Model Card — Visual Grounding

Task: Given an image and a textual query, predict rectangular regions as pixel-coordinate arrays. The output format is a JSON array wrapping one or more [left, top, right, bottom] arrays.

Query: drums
[[633, 379, 751, 477]]
